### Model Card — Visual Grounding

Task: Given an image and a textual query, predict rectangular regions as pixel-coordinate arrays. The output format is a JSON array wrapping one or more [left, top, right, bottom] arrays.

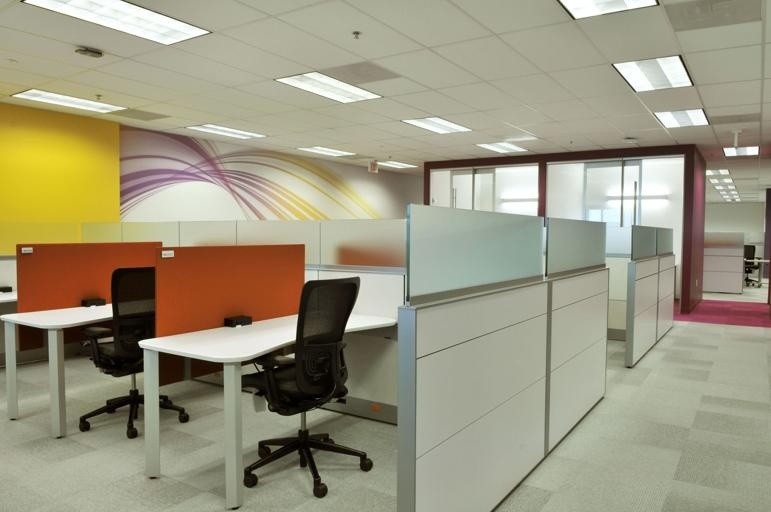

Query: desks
[[0, 304, 113, 439], [138, 315, 397, 510], [745, 259, 770, 288]]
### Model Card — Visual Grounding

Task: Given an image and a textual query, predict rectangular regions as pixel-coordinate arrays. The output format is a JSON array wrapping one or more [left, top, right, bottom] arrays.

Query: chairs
[[80, 298, 112, 367], [744, 245, 760, 286], [242, 278, 373, 497], [79, 267, 188, 438]]
